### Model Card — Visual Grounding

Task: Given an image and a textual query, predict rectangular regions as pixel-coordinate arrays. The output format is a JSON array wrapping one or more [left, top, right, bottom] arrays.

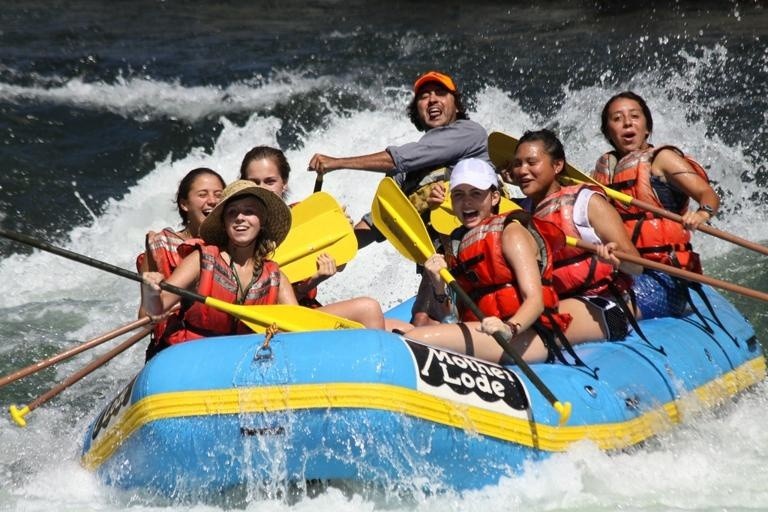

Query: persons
[[587, 91, 720, 322], [510, 129, 643, 346], [397, 159, 551, 366], [307, 72, 499, 333], [139, 180, 299, 344], [136, 169, 228, 363], [237, 146, 386, 331]]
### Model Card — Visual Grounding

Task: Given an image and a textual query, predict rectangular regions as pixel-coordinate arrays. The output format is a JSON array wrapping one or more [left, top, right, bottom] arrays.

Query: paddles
[[430, 181, 768, 300], [9, 191, 344, 427], [487, 132, 768, 253], [371, 176, 571, 425], [0, 211, 361, 387], [0, 227, 366, 334], [307, 165, 334, 192]]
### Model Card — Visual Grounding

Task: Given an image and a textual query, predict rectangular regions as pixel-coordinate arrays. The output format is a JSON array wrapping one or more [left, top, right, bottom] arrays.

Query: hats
[[447, 155, 502, 197], [413, 69, 459, 98], [195, 177, 296, 250]]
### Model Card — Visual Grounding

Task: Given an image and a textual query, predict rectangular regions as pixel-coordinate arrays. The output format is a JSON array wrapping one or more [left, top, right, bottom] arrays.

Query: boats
[[76, 281, 766, 502]]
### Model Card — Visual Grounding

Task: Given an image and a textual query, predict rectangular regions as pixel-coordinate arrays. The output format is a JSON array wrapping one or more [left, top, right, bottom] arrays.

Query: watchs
[[696, 202, 716, 219]]
